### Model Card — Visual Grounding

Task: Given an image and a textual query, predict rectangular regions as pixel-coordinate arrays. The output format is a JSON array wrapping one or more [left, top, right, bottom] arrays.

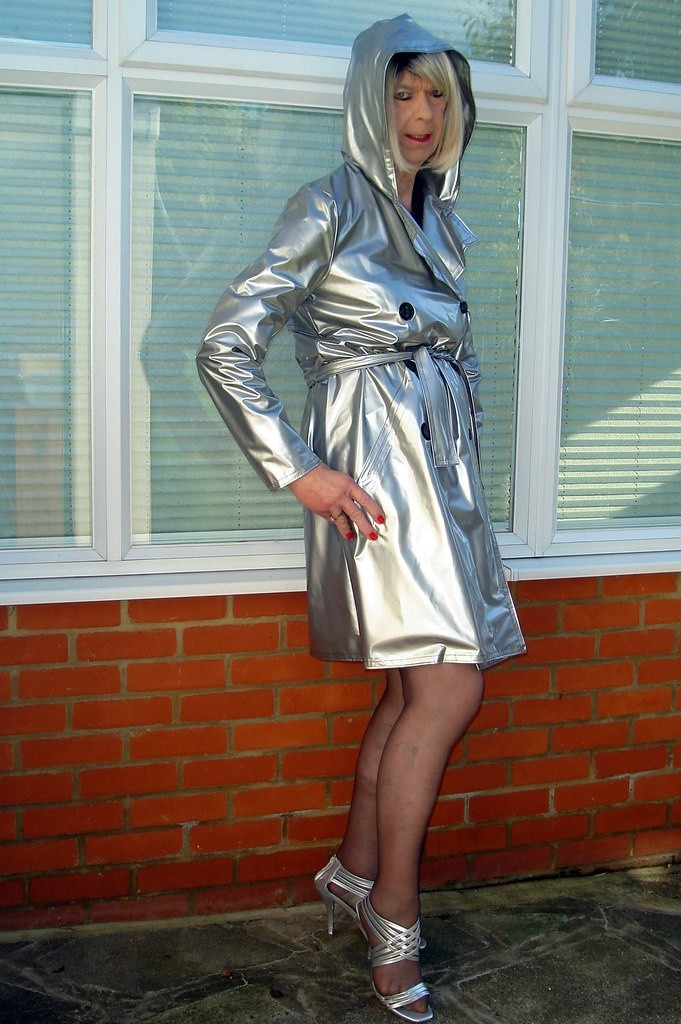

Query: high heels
[[314, 854, 376, 944], [355, 892, 435, 1023]]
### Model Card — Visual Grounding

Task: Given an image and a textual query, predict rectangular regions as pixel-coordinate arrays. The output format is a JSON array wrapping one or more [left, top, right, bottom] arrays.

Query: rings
[[328, 512, 345, 522]]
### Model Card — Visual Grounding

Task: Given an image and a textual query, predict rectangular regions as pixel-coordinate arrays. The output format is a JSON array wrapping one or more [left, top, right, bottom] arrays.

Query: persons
[[194, 11, 529, 1023]]
